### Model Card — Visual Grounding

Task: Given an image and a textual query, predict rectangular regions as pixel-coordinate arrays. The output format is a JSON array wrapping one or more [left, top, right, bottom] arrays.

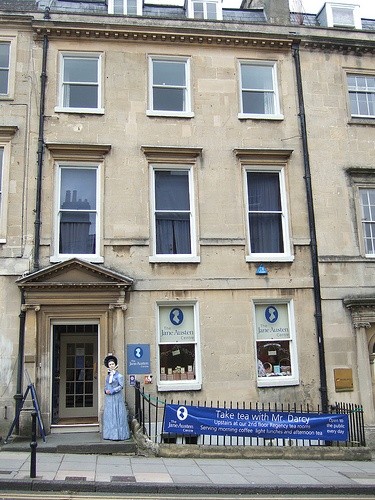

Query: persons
[[101, 356, 131, 440]]
